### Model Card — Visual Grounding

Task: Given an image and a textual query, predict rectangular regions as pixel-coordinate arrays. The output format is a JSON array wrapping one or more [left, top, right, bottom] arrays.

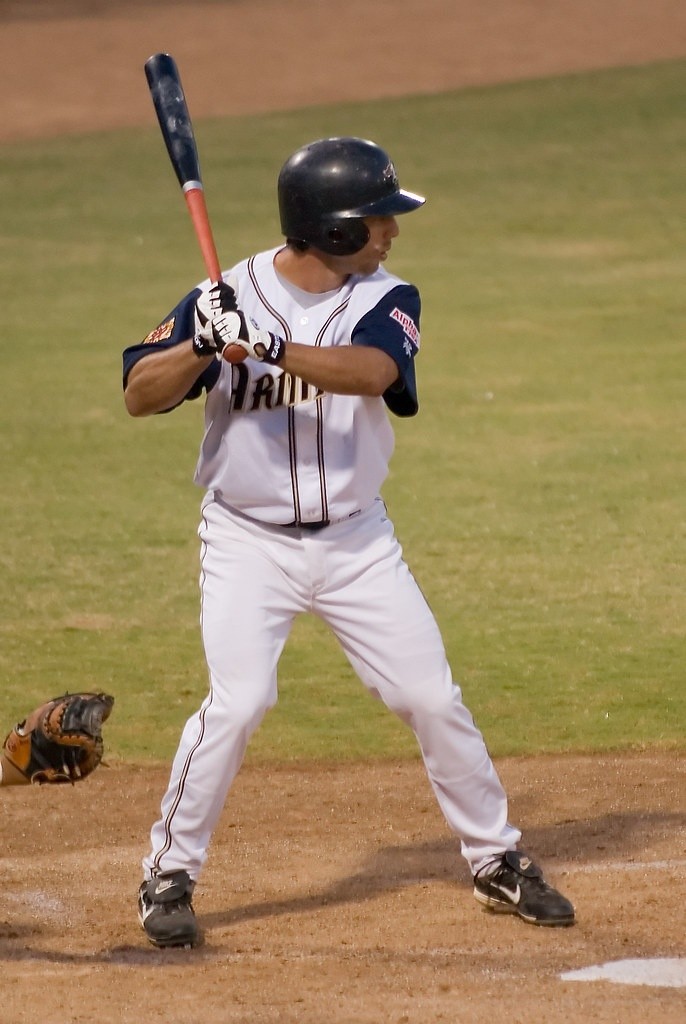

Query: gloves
[[190, 282, 241, 355], [197, 310, 284, 365]]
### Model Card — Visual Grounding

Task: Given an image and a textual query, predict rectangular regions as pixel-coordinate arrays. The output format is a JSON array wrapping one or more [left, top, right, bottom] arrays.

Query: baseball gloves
[[2, 689, 116, 788]]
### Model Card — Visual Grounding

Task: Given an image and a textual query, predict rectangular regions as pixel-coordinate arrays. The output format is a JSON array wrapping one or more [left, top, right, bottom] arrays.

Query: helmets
[[278, 134, 430, 257]]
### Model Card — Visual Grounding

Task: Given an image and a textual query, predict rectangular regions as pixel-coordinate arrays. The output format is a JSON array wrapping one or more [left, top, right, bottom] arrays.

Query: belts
[[280, 520, 331, 531]]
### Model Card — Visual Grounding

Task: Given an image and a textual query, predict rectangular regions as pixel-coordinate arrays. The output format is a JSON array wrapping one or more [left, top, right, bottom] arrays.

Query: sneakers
[[138, 872, 200, 946], [470, 847, 577, 926]]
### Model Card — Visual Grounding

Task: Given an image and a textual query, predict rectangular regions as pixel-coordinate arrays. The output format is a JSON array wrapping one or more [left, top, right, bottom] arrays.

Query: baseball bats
[[143, 51, 248, 366]]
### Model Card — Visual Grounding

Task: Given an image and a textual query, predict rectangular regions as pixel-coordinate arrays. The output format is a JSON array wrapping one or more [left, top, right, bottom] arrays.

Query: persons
[[123, 137, 577, 947], [0, 689, 113, 785]]
